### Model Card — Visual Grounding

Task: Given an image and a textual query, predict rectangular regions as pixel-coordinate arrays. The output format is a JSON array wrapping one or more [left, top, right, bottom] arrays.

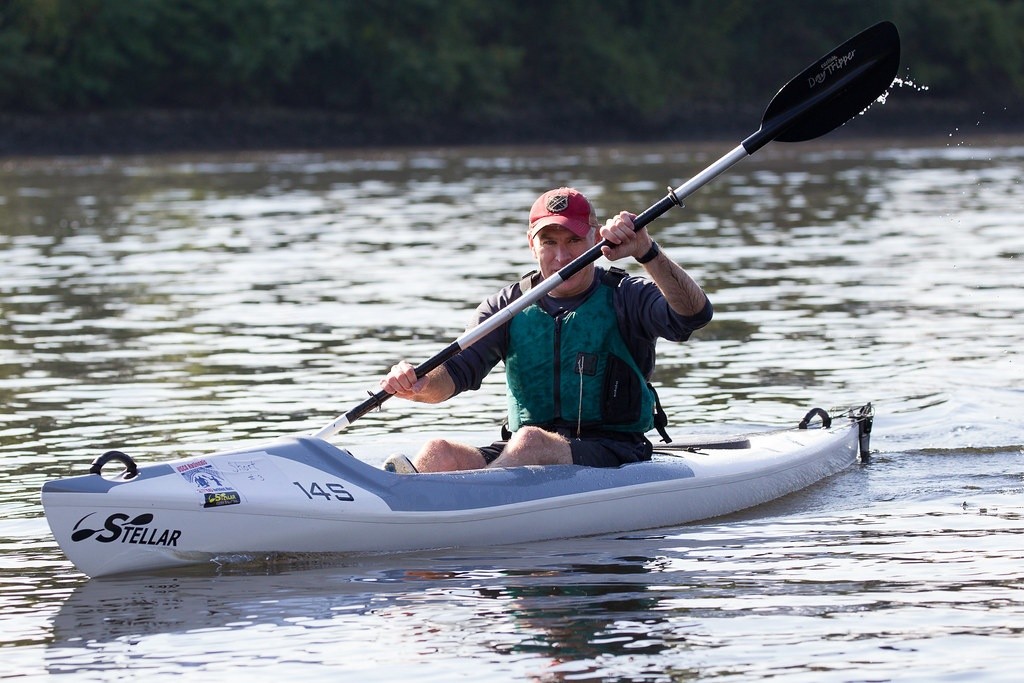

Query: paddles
[[306, 17, 905, 445]]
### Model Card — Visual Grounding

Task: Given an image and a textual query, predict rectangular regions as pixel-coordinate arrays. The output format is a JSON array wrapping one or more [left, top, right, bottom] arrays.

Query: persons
[[380, 187, 713, 475]]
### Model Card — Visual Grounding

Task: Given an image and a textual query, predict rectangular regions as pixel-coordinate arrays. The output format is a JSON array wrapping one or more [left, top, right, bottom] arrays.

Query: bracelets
[[636, 237, 659, 265]]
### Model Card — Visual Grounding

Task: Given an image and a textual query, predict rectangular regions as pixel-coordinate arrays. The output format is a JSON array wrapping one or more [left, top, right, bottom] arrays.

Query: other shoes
[[381, 453, 420, 474]]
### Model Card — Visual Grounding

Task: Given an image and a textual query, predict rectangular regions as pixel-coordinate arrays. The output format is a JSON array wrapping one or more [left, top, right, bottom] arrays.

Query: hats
[[529, 188, 599, 239]]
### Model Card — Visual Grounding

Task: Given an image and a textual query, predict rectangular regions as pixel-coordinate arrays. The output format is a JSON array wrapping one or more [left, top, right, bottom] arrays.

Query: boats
[[40, 401, 878, 580]]
